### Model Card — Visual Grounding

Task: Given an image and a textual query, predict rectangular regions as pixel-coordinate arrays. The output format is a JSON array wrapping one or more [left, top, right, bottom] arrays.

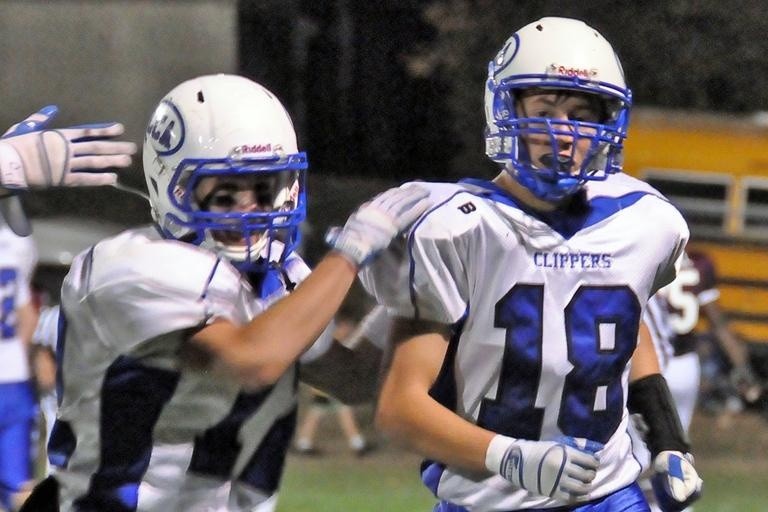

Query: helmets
[[139, 71, 303, 266], [481, 16, 630, 178]]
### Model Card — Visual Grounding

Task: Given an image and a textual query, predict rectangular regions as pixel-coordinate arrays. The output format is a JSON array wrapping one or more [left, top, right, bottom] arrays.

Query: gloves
[[323, 183, 435, 276], [648, 449, 705, 511], [484, 433, 605, 506]]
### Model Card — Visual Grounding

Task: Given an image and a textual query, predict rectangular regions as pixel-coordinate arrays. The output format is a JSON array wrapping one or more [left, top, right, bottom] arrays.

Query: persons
[[290, 314, 373, 459], [632, 238, 761, 438], [2, 225, 64, 506], [12, 68, 434, 510], [370, 13, 708, 512]]
